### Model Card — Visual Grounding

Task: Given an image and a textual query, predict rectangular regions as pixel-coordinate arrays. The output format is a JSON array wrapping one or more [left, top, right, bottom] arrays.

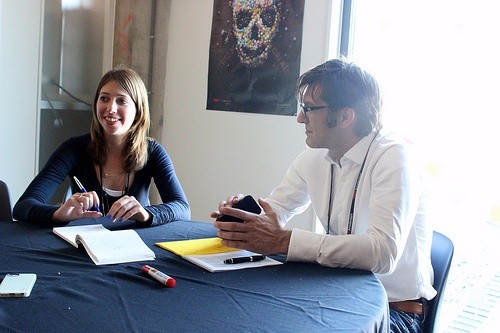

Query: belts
[[389, 301, 423, 315]]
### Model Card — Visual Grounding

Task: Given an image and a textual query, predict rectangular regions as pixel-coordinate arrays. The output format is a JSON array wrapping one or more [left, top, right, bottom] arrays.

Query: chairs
[[423, 230, 454, 333]]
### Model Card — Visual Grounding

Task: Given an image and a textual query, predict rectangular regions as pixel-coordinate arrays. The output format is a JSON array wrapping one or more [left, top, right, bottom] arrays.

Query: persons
[[209, 59, 437, 333], [12, 68, 192, 227]]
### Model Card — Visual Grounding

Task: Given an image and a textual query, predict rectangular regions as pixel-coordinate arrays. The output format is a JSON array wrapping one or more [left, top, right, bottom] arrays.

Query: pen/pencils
[[223, 254, 267, 264], [73, 176, 101, 212]]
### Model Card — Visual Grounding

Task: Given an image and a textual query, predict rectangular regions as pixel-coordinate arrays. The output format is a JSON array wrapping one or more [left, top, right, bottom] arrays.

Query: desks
[[0, 216, 390, 333]]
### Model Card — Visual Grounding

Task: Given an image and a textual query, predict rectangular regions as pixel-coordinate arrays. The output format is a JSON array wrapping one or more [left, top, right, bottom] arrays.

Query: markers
[[142, 265, 176, 288]]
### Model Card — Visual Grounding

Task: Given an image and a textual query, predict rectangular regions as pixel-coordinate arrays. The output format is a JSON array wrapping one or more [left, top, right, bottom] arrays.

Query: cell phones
[[216, 195, 261, 231]]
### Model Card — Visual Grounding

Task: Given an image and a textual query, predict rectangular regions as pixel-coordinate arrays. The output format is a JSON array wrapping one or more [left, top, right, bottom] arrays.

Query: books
[[155, 237, 283, 272], [52, 224, 157, 265]]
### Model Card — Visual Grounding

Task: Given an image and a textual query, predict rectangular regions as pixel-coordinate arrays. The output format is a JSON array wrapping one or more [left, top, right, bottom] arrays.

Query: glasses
[[300, 104, 330, 117]]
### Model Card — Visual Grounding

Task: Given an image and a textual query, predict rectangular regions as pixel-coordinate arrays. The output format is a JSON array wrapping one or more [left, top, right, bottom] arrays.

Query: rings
[[76, 194, 83, 200]]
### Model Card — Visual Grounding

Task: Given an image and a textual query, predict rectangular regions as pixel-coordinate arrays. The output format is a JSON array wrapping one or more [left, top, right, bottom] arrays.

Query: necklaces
[[103, 170, 108, 177]]
